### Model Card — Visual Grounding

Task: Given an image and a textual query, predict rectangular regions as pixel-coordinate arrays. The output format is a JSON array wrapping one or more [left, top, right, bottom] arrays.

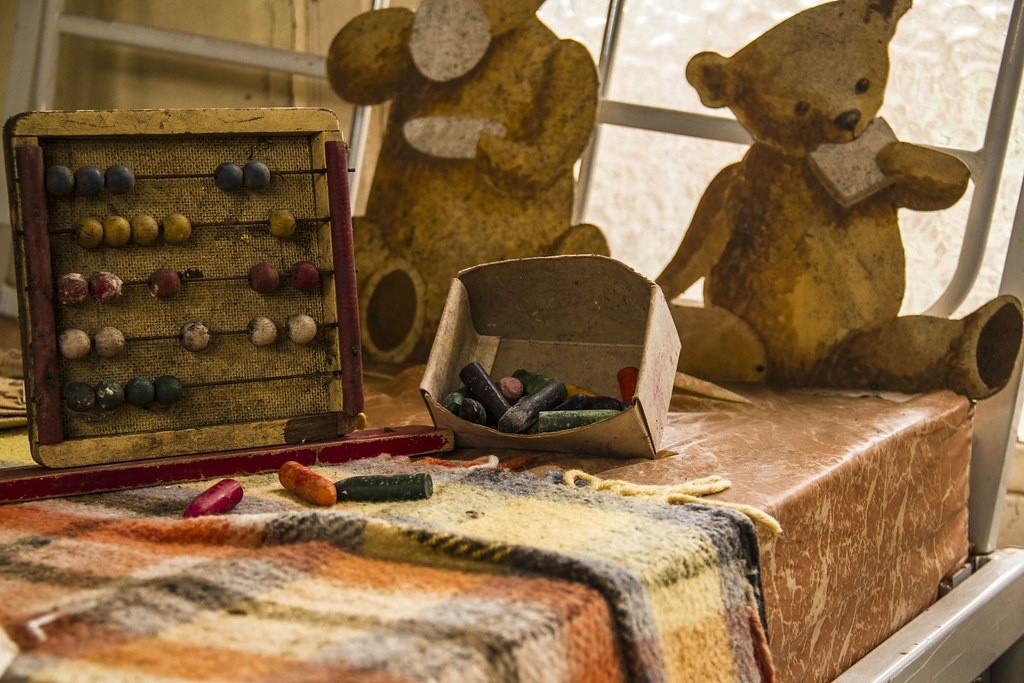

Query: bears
[[326, 0, 611, 366], [647, 0, 1024, 400]]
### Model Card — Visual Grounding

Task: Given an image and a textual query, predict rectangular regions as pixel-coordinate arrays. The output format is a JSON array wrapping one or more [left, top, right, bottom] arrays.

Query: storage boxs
[[418, 253, 681, 459]]
